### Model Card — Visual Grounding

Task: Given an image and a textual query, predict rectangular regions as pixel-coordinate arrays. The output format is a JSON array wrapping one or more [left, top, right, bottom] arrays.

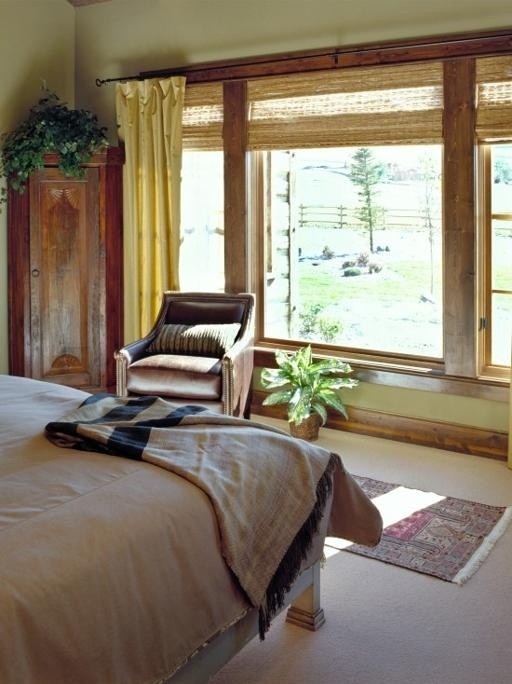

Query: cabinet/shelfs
[[7, 147, 125, 395]]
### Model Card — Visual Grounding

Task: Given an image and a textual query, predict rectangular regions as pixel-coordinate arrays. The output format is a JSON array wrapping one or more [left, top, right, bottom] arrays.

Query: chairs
[[114, 291, 256, 419]]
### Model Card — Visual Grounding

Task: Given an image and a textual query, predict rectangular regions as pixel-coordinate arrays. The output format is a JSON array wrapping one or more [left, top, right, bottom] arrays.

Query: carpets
[[324, 473, 512, 587]]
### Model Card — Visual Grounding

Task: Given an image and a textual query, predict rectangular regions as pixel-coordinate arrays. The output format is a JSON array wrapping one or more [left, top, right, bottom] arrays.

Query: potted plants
[[259, 343, 359, 441]]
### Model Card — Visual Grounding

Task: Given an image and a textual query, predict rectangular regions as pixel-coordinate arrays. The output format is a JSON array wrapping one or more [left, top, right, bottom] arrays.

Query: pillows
[[146, 323, 241, 355]]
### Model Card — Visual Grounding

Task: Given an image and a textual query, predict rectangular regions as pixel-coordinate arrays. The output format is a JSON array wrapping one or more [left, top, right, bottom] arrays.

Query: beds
[[0, 374, 382, 684]]
